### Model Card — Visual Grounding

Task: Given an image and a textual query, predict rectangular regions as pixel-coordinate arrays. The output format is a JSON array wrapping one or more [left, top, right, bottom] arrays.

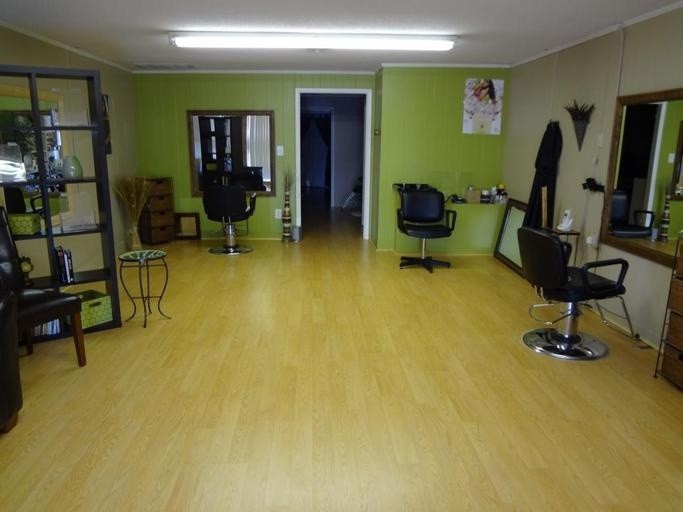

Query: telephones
[[561, 207, 573, 231]]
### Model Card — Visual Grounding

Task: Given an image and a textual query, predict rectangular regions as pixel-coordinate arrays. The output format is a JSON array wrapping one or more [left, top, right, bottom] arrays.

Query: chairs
[[229, 165, 267, 191], [201, 183, 258, 255], [395, 188, 456, 273], [607, 190, 656, 239], [0, 206, 86, 366], [0, 287, 23, 434], [4, 186, 45, 219], [513, 224, 636, 361]]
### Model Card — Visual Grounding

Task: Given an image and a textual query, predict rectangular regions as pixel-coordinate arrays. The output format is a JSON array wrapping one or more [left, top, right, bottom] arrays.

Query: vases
[[126, 217, 143, 252], [658, 193, 669, 241], [279, 185, 294, 243], [224, 142, 231, 154]]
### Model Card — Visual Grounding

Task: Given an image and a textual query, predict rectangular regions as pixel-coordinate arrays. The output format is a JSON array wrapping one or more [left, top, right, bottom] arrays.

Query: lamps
[[563, 99, 595, 152], [166, 30, 459, 51]]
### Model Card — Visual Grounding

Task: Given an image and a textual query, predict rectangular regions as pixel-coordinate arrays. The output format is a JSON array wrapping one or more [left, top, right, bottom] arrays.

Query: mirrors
[[493, 198, 529, 279], [0, 82, 75, 233], [669, 117, 682, 203], [598, 87, 682, 271], [187, 108, 277, 198]]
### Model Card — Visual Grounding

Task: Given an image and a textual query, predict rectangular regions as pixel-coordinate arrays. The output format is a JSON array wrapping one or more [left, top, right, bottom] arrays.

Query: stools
[[117, 248, 172, 327]]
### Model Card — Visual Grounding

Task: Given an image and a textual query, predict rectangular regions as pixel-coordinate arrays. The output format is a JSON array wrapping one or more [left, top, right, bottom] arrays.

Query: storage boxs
[[63, 289, 113, 329], [206, 162, 217, 171], [8, 211, 40, 236]]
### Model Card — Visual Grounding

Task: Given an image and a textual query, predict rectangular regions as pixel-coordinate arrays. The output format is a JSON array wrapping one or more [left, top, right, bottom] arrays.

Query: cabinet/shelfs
[[197, 115, 246, 191], [141, 175, 174, 245], [652, 235, 682, 394], [0, 64, 121, 345]]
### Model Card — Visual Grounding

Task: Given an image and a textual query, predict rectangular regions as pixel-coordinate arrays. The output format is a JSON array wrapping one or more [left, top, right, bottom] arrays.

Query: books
[[32, 289, 60, 337], [55, 245, 74, 283]]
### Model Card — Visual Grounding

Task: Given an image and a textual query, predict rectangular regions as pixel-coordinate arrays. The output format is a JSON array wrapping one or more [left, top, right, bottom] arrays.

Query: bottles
[[480, 184, 506, 203]]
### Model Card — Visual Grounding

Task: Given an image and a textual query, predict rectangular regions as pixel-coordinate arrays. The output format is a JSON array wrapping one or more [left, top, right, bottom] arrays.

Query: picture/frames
[[175, 212, 201, 240]]
